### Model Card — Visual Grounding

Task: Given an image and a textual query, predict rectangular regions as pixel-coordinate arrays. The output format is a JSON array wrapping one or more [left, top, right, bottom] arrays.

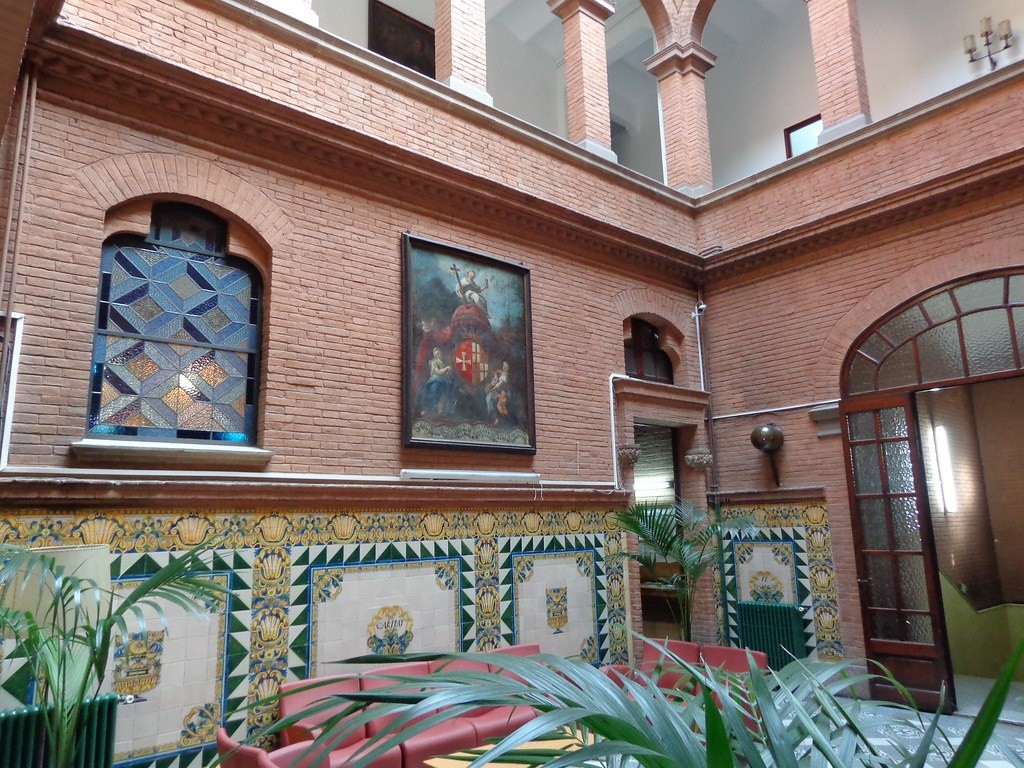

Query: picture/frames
[[368, 0, 436, 80], [402, 232, 539, 457]]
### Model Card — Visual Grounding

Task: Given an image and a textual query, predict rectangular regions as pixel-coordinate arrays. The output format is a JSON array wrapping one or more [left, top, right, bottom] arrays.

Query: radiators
[[0, 692, 135, 768], [736, 601, 807, 691]]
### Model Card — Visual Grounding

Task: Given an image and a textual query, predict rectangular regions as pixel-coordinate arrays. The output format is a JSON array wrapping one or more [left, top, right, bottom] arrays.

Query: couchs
[[216, 644, 577, 768], [599, 639, 768, 745]]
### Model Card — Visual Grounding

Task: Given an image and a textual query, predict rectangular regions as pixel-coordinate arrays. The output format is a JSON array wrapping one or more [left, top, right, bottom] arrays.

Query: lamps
[[963, 16, 1013, 71]]
